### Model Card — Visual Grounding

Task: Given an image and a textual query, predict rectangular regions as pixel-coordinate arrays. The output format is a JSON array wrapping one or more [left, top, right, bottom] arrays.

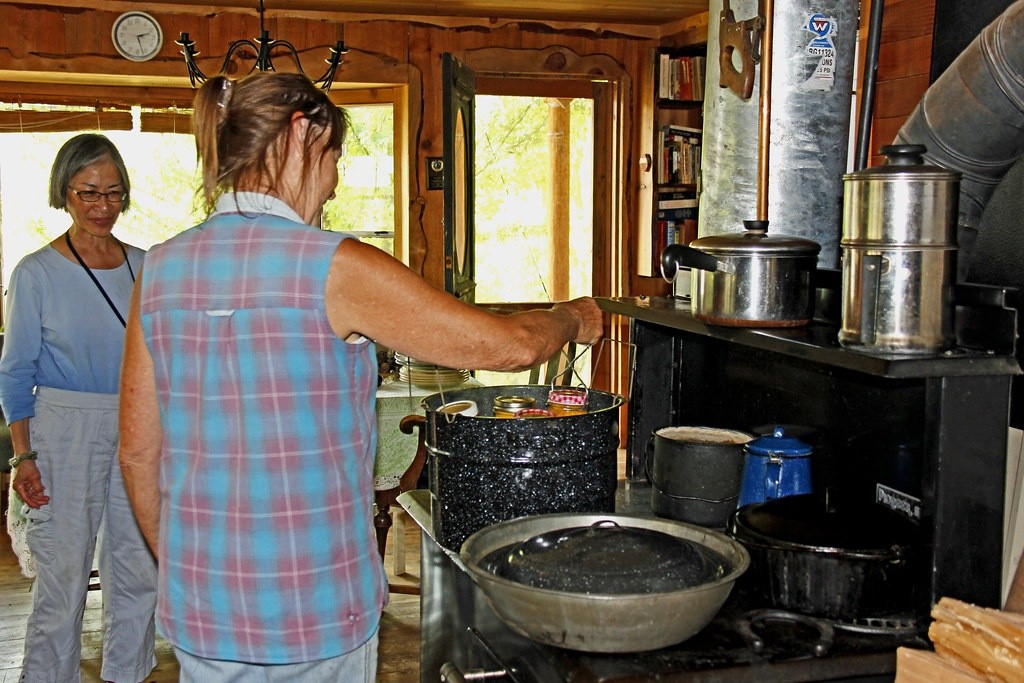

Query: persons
[[119, 72, 602, 683], [0, 134, 159, 683]]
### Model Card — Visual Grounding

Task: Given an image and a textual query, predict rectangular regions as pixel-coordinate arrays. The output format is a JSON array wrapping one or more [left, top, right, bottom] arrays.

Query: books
[[656, 125, 702, 200], [659, 54, 706, 101], [656, 208, 698, 273]]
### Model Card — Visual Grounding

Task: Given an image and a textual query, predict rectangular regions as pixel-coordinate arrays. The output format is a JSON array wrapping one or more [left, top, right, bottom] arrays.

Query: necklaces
[[66, 228, 135, 328]]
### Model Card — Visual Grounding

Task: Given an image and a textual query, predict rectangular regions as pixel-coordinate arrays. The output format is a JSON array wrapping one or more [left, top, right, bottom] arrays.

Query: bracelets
[[9, 450, 38, 467]]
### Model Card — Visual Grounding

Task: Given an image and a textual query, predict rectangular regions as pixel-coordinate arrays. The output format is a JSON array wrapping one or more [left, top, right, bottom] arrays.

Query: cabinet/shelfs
[[631, 46, 708, 278]]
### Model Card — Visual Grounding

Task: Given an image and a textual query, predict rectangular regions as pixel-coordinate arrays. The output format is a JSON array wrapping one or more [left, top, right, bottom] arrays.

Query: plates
[[394, 351, 470, 387]]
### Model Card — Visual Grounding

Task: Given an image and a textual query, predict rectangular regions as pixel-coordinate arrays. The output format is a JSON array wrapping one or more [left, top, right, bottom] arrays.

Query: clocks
[[112, 11, 164, 63]]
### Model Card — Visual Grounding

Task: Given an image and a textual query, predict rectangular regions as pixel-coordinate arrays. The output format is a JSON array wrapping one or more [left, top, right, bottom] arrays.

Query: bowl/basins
[[459, 512, 751, 653]]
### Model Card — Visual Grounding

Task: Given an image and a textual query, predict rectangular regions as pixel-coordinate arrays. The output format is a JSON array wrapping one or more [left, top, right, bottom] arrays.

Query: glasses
[[67, 184, 129, 202]]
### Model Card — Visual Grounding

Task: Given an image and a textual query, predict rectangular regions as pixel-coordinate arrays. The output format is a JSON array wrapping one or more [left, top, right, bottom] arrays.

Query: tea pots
[[737, 427, 814, 510]]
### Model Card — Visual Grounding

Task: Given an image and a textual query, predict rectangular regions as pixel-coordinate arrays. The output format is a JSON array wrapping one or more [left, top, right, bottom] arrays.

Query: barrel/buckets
[[421, 384, 625, 552], [645, 426, 756, 530]]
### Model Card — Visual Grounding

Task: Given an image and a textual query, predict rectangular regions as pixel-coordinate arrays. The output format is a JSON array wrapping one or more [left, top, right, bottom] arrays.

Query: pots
[[661, 220, 822, 328], [839, 142, 964, 355], [727, 493, 914, 620]]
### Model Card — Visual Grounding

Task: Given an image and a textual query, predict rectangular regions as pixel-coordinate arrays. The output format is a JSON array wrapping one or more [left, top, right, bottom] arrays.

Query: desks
[[5, 371, 485, 590]]
